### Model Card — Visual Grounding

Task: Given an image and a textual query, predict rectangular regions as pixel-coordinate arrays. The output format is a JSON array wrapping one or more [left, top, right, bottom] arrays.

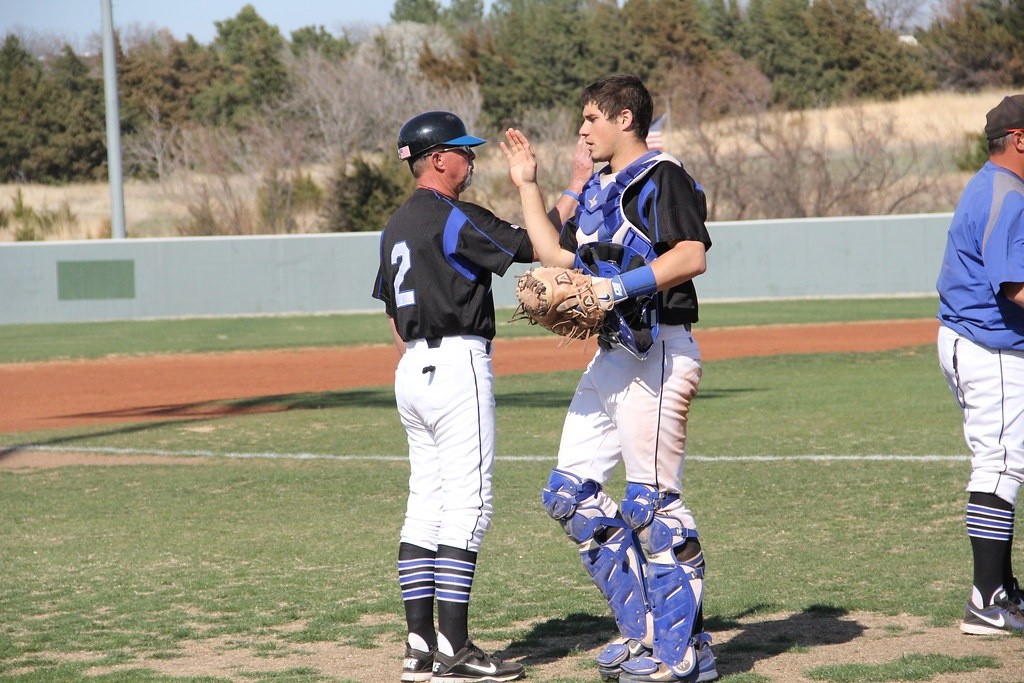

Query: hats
[[398, 112, 487, 161], [985, 95, 1023, 140]]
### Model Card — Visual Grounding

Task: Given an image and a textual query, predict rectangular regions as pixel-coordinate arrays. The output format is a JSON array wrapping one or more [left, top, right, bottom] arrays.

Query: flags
[[645, 113, 666, 151]]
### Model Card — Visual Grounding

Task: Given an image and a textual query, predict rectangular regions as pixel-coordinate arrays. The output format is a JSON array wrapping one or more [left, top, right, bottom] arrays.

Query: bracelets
[[562, 190, 580, 201]]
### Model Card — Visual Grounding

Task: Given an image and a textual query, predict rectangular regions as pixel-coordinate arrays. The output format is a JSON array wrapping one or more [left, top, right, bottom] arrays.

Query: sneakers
[[597, 640, 719, 683], [401, 638, 526, 683], [963, 588, 1024, 635]]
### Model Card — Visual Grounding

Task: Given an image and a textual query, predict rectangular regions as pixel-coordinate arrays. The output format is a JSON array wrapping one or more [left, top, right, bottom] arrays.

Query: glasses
[[423, 145, 471, 159]]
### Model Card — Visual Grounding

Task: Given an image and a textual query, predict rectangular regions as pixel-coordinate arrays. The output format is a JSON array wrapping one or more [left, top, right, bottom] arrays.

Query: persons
[[499, 75, 718, 683], [936, 95, 1024, 635], [373, 111, 593, 683]]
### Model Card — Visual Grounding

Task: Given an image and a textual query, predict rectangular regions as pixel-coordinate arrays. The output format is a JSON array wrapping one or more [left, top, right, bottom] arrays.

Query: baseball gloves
[[516, 265, 615, 339]]
